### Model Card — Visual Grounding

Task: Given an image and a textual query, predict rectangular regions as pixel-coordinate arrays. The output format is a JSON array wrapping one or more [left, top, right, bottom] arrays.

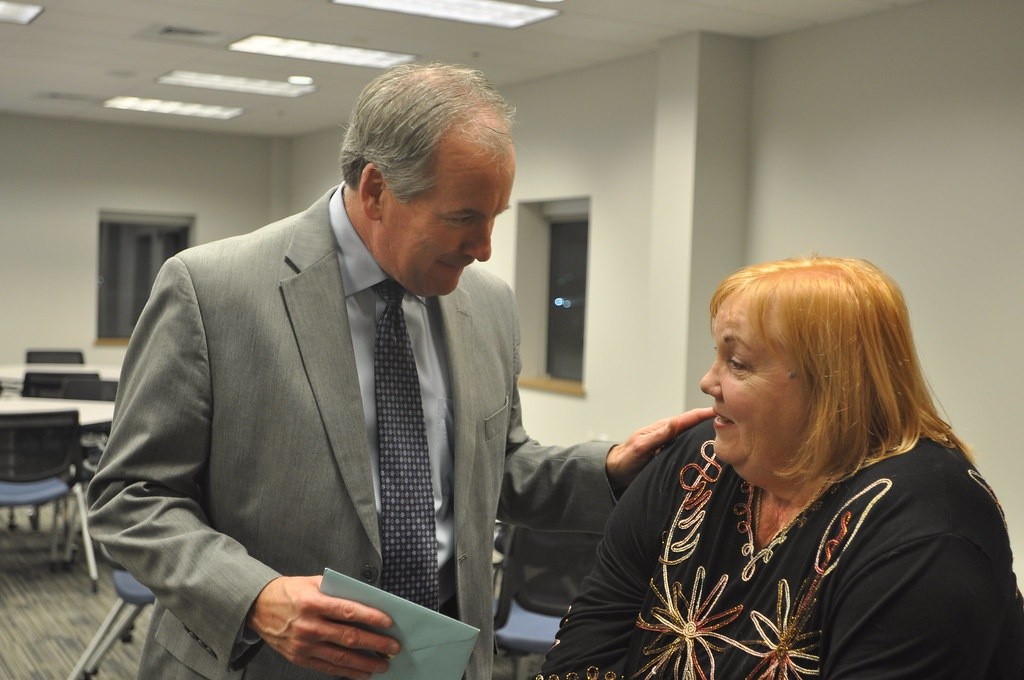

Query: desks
[[0, 396, 114, 431]]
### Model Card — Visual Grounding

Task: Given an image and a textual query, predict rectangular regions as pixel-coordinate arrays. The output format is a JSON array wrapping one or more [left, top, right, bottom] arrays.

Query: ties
[[370, 276, 440, 617]]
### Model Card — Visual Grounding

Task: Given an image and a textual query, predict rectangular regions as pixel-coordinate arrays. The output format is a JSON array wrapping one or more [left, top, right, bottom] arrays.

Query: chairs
[[485, 525, 603, 680], [0, 350, 157, 680]]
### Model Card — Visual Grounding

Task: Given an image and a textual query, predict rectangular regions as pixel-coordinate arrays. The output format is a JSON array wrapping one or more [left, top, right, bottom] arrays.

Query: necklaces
[[756, 473, 831, 539]]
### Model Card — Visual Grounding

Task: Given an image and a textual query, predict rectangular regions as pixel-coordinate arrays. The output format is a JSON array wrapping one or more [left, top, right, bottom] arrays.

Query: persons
[[530, 256, 1024, 680], [86, 62, 714, 680]]
